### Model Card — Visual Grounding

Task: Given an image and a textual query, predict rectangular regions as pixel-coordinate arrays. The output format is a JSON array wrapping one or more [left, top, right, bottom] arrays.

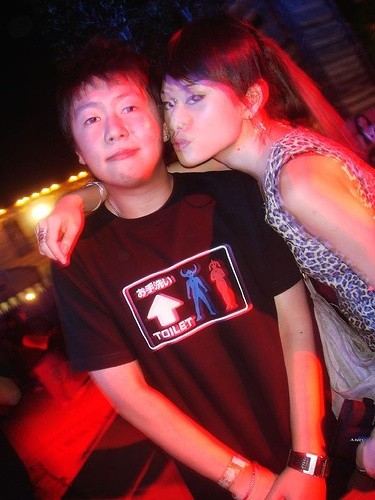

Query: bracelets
[[287, 449, 334, 478], [241, 461, 256, 500], [81, 182, 105, 211], [219, 452, 250, 490]]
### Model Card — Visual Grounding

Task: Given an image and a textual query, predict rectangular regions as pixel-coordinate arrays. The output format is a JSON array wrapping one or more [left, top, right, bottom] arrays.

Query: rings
[[37, 228, 46, 245], [354, 458, 367, 474]]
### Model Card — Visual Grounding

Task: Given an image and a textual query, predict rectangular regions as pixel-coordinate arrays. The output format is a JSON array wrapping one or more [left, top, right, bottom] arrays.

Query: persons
[[49, 36, 293, 366], [33, 12, 375, 480]]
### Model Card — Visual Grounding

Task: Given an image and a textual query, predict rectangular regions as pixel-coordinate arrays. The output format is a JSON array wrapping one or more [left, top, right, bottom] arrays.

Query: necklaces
[[256, 176, 267, 208], [106, 197, 124, 219]]
